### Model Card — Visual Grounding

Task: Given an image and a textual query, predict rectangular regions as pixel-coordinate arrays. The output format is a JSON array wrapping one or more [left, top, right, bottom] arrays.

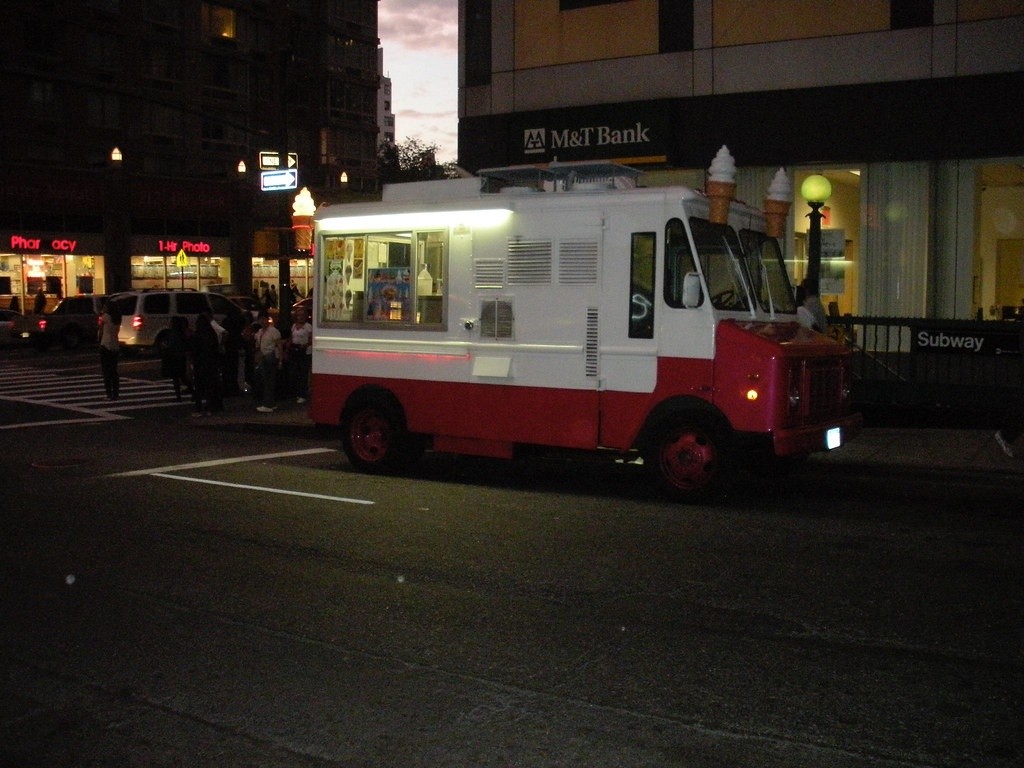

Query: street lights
[[802, 173, 832, 296]]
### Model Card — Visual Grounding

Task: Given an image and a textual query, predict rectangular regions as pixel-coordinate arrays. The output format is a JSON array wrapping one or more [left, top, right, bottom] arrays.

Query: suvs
[[99, 287, 266, 359]]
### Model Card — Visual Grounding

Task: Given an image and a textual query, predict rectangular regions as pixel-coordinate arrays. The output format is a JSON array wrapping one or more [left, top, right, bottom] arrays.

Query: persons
[[55, 293, 63, 305], [8, 296, 20, 312], [166, 285, 313, 417], [801, 278, 826, 333], [99, 301, 121, 400], [33, 287, 47, 315]]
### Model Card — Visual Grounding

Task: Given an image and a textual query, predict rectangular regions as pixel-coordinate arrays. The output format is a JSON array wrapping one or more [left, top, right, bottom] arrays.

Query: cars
[[26, 294, 110, 350], [0, 309, 35, 348], [200, 284, 312, 324]]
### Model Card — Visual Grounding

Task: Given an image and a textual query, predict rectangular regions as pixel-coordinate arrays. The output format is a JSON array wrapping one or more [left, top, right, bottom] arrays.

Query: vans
[[310, 143, 863, 506]]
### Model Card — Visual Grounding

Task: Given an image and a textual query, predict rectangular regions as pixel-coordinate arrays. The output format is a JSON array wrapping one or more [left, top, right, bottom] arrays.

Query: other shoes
[[297, 396, 307, 403], [256, 406, 278, 412], [113, 397, 117, 400], [192, 410, 211, 417], [102, 397, 112, 400]]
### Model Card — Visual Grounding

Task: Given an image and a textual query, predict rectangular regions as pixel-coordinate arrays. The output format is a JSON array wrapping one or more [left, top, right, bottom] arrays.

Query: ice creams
[[345, 245, 352, 310], [706, 144, 736, 223], [764, 166, 793, 238], [291, 187, 316, 250]]
